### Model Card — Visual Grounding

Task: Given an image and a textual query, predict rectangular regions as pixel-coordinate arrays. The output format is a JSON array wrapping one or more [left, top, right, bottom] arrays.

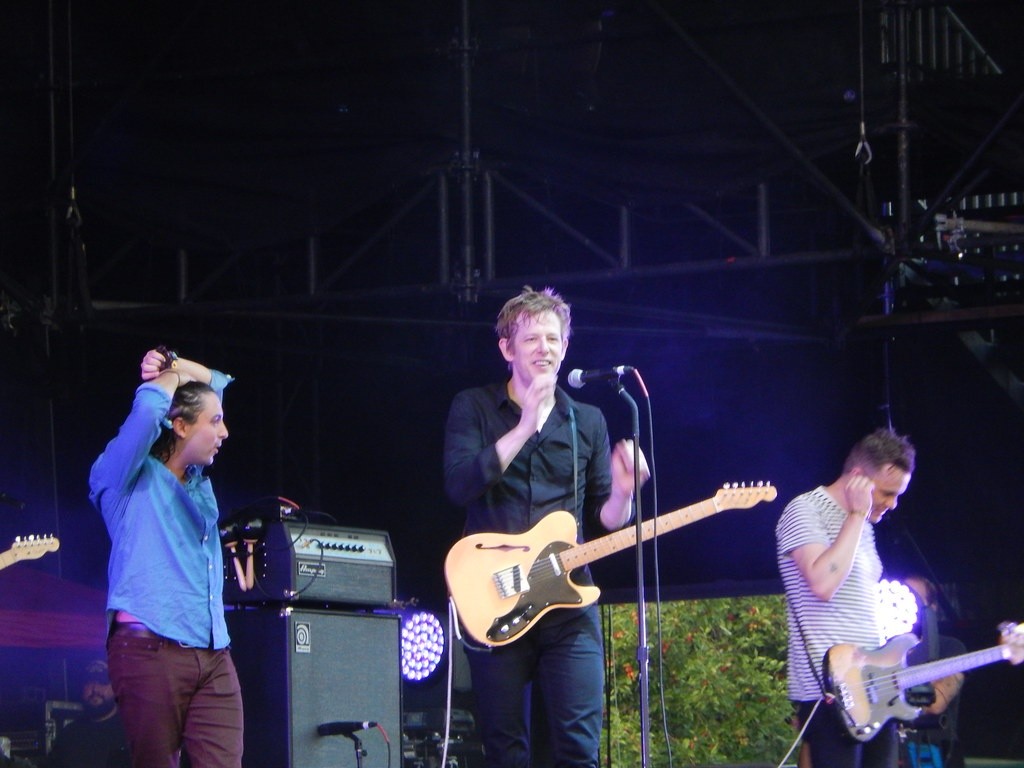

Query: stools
[[908, 742, 943, 768]]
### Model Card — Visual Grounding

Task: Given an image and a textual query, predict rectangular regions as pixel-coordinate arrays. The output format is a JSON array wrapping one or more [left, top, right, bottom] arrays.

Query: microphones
[[568, 365, 634, 389]]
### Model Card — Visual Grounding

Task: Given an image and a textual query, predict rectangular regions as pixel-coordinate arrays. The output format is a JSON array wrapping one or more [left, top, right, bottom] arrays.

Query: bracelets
[[156, 346, 178, 371]]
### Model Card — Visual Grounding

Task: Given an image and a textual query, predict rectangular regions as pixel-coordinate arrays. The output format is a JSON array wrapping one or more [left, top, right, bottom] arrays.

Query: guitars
[[0, 533, 61, 571], [822, 621, 1024, 745], [443, 480, 778, 649]]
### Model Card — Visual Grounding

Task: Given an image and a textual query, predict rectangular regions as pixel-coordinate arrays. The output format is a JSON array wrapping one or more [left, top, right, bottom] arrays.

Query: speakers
[[220, 522, 405, 768]]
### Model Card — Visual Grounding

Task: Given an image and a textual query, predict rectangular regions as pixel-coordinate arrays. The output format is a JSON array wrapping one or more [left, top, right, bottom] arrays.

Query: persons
[[772, 429, 918, 766], [421, 283, 653, 767], [891, 573, 972, 766], [85, 341, 250, 768]]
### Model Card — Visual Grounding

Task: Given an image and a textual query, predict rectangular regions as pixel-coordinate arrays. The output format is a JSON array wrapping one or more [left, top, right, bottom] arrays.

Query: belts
[[113, 626, 198, 648]]
